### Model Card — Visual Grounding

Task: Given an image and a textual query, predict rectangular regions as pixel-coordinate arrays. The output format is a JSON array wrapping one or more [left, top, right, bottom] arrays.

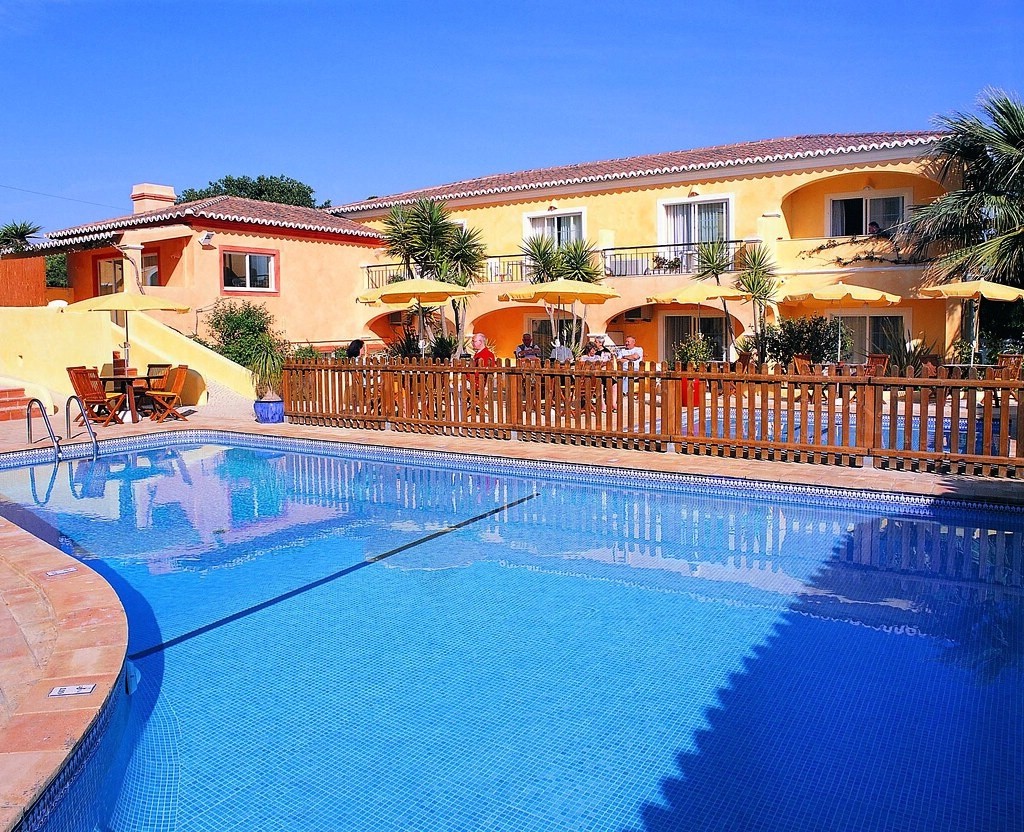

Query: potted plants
[[249, 346, 286, 423]]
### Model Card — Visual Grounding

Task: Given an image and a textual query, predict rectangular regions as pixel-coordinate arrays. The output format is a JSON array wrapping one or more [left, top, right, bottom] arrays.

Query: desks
[[542, 366, 576, 413], [808, 363, 868, 404], [689, 361, 725, 393], [89, 374, 163, 421], [942, 364, 1005, 407], [392, 370, 442, 418]]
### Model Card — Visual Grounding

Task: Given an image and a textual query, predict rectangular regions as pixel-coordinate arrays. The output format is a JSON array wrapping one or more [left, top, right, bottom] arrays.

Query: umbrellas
[[497, 280, 621, 358], [57, 292, 191, 405], [356, 279, 485, 358], [646, 282, 752, 333], [920, 278, 1024, 365], [783, 281, 902, 362]]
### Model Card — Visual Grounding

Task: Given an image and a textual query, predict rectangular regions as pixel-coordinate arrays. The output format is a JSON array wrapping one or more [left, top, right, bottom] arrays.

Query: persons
[[551, 338, 575, 400], [517, 333, 541, 400], [466, 333, 495, 398], [593, 336, 612, 357], [869, 221, 890, 239], [617, 336, 643, 397], [581, 341, 617, 413], [348, 341, 406, 419]]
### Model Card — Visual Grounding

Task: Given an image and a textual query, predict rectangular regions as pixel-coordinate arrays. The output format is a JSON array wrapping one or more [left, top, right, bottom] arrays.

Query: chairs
[[919, 355, 954, 399], [717, 351, 753, 398], [520, 359, 603, 418], [981, 354, 1023, 403], [791, 354, 828, 403], [66, 363, 188, 427], [849, 354, 892, 404], [349, 364, 499, 418]]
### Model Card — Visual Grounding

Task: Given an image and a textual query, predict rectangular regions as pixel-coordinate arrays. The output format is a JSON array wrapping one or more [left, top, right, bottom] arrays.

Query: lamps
[[688, 188, 700, 196], [862, 185, 874, 191], [548, 202, 557, 211]]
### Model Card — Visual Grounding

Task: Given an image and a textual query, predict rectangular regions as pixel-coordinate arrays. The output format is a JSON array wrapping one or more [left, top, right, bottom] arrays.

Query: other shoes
[[404, 408, 420, 415], [602, 409, 607, 413], [466, 406, 472, 417], [612, 409, 617, 413]]
